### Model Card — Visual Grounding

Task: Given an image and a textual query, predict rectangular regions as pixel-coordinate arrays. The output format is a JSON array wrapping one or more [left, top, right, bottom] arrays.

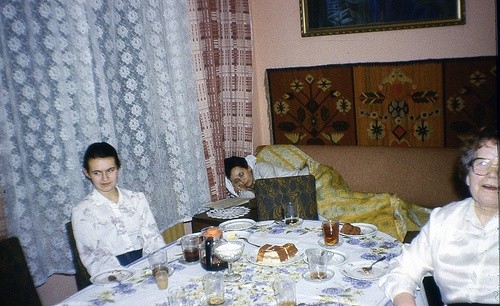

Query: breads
[[256, 243, 298, 263], [341, 223, 361, 235]]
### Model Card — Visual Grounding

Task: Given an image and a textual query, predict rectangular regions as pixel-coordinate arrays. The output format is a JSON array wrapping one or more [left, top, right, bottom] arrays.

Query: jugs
[[199, 226, 228, 271]]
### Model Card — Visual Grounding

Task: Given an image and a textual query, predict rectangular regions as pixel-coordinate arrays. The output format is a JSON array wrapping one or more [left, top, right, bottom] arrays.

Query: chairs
[[0, 233, 43, 306], [254, 175, 320, 222], [65, 222, 92, 291]]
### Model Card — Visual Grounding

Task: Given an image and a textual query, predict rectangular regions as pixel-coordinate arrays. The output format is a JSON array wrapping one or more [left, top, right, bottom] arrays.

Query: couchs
[[246, 145, 471, 243]]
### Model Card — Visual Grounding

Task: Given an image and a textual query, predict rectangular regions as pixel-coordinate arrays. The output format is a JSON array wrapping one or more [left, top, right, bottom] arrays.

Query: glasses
[[467, 157, 500, 178]]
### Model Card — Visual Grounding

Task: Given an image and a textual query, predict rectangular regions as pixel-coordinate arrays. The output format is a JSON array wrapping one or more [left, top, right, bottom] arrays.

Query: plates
[[318, 236, 344, 248], [199, 295, 233, 306], [219, 219, 256, 231], [339, 221, 379, 240], [319, 248, 350, 267], [341, 260, 391, 281], [178, 255, 200, 265], [89, 268, 134, 287], [146, 266, 175, 277], [302, 269, 335, 282]]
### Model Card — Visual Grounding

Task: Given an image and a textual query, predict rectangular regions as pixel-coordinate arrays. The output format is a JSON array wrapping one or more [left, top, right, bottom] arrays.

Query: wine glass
[[148, 250, 169, 274], [215, 241, 245, 281]]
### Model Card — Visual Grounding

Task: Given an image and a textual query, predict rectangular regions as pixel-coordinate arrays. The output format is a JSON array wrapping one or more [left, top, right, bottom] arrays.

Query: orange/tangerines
[[203, 228, 220, 237]]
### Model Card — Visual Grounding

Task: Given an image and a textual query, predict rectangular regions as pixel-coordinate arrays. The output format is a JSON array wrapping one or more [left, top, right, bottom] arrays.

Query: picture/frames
[[299, 0, 468, 38]]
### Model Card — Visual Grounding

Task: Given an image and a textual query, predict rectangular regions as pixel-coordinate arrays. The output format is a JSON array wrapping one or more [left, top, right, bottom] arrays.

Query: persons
[[223, 156, 406, 222], [71, 142, 167, 277], [378, 125, 500, 306]]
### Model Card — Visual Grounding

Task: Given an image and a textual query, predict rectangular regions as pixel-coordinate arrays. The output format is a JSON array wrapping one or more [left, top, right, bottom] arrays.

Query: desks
[[192, 201, 253, 233]]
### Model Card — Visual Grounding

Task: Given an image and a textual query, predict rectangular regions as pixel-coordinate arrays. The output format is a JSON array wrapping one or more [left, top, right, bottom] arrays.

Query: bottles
[[284, 202, 299, 233]]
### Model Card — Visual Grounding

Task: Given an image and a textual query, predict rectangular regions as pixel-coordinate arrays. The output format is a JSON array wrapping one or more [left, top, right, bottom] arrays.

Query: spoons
[[108, 275, 128, 287], [362, 256, 388, 270]]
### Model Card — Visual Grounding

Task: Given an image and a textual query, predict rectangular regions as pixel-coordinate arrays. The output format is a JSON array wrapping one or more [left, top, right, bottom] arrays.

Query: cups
[[153, 270, 169, 290], [306, 248, 328, 279], [274, 276, 297, 306], [203, 273, 225, 305], [322, 219, 340, 246], [180, 235, 200, 262]]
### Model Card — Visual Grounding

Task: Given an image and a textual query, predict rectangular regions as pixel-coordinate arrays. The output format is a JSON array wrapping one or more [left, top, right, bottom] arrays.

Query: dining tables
[[48, 220, 428, 306]]
